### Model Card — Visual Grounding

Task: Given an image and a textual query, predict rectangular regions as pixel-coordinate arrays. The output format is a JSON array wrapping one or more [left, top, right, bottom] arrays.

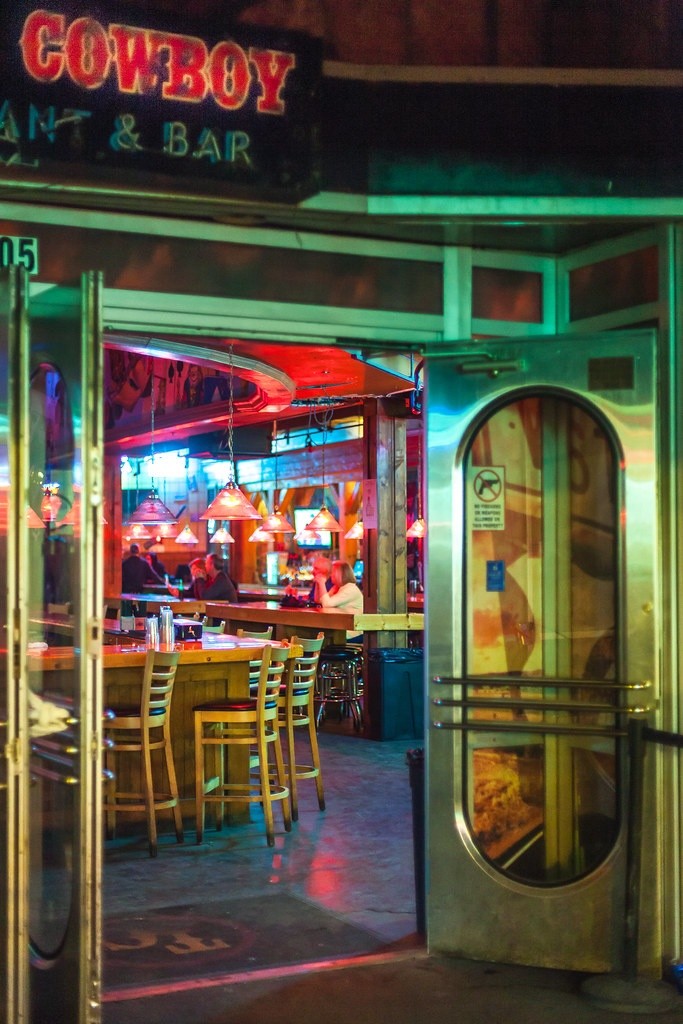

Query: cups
[[370, 507, 374, 516]]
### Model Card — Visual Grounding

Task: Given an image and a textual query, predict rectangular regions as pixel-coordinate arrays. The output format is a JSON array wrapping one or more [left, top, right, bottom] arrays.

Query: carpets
[[37, 889, 399, 989]]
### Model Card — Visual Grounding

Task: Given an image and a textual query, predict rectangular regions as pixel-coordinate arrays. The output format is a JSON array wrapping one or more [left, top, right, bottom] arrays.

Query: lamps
[[198, 346, 262, 522], [345, 501, 363, 539], [210, 521, 235, 544], [262, 417, 295, 534], [152, 476, 178, 539], [249, 526, 275, 543], [122, 462, 154, 539], [407, 414, 426, 539], [129, 361, 175, 527], [308, 391, 342, 532], [175, 456, 198, 544]]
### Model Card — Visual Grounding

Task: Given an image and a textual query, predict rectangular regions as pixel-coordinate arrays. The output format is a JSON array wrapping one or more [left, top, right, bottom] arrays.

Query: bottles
[[178, 579, 183, 601], [159, 605, 174, 652], [120, 599, 134, 633], [146, 615, 160, 653], [366, 497, 371, 516], [133, 601, 147, 637]]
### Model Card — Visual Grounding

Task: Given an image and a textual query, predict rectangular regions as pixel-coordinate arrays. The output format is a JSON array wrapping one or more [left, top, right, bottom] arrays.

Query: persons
[[285, 557, 332, 607], [314, 561, 363, 644], [122, 543, 165, 594], [195, 554, 237, 604], [167, 558, 207, 600], [148, 552, 164, 578]]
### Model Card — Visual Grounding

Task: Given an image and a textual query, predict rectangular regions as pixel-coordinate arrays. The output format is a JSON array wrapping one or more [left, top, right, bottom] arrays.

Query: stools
[[104, 650, 184, 856], [194, 621, 364, 845]]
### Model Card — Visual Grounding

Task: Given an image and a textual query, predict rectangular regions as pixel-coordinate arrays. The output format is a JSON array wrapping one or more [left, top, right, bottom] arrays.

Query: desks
[[206, 601, 424, 640], [120, 591, 230, 616]]
